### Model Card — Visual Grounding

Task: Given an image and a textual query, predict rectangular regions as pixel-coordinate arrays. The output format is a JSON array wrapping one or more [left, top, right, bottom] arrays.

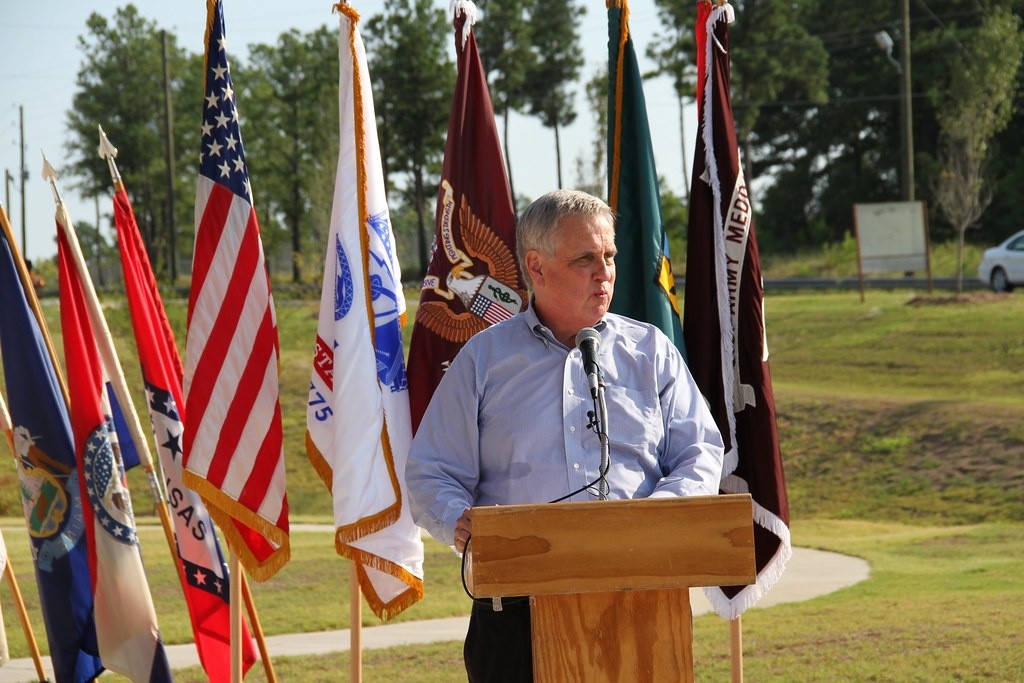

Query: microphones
[[574, 327, 602, 400]]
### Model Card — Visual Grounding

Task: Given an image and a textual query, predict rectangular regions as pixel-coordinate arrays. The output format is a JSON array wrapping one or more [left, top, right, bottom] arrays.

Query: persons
[[405, 190, 725, 683]]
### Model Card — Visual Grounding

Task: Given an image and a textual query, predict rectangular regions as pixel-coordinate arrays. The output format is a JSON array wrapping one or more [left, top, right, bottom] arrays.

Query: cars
[[976, 229, 1024, 293]]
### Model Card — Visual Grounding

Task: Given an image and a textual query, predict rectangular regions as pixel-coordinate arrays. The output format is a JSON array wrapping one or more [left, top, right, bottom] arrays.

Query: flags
[[306, 0, 790, 622], [0, 0, 293, 682]]
